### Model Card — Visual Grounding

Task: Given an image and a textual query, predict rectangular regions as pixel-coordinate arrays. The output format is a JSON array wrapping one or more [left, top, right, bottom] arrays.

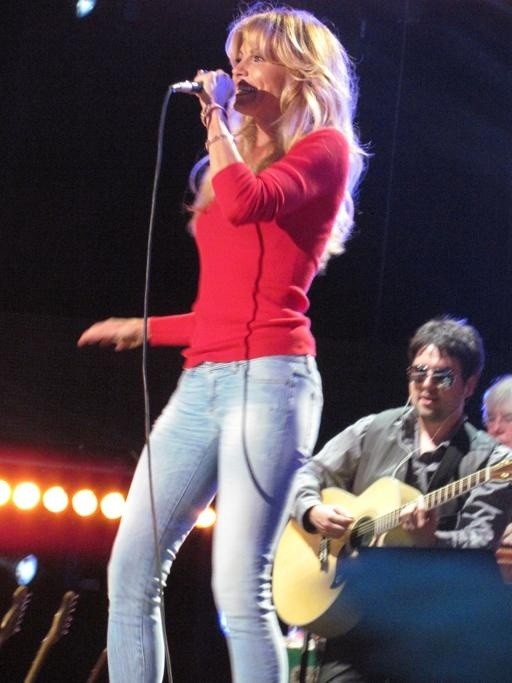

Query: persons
[[75, 4, 376, 682], [287, 311, 512, 682], [480, 370, 512, 550]]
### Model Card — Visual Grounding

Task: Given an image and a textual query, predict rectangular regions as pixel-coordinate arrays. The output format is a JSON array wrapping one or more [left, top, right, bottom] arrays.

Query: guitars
[[270, 453, 512, 638]]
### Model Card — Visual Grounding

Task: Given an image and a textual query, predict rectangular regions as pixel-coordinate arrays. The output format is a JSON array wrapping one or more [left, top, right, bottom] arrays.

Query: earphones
[[463, 385, 469, 395]]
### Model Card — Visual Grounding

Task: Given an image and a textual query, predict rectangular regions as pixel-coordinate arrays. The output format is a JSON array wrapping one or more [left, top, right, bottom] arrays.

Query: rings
[[196, 68, 208, 75], [405, 517, 413, 523]]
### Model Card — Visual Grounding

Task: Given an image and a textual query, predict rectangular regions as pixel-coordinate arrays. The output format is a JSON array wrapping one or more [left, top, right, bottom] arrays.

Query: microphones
[[172, 80, 203, 92]]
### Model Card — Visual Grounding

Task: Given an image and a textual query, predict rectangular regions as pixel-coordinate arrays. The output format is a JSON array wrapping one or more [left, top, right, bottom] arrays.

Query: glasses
[[405, 365, 459, 392]]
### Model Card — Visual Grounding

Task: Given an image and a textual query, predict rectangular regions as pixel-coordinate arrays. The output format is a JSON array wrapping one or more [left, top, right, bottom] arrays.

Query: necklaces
[[240, 130, 283, 173]]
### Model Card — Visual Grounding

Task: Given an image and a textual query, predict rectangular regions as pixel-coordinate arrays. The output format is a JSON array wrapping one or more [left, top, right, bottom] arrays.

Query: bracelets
[[204, 132, 233, 149], [199, 103, 228, 125]]
